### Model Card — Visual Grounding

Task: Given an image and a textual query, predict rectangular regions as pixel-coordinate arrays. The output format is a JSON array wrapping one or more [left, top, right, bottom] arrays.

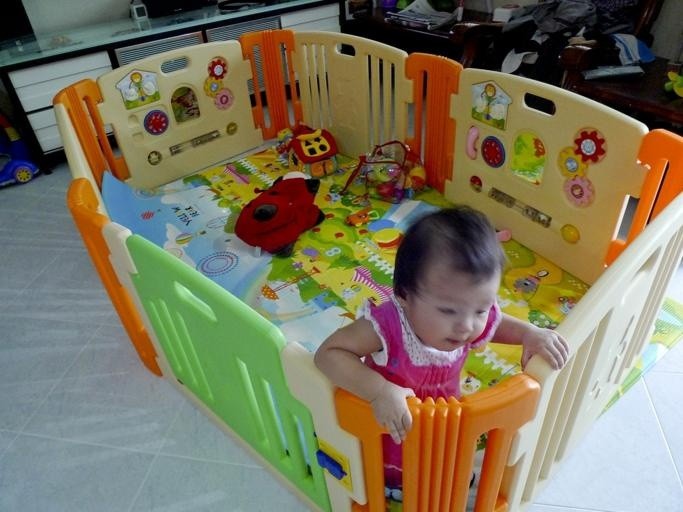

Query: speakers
[[130, 2, 149, 20]]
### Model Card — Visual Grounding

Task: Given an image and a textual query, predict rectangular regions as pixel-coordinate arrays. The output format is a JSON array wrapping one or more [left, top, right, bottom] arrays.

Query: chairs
[[447, 1, 663, 98]]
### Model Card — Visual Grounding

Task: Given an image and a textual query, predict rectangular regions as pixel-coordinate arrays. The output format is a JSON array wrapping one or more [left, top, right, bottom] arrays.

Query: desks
[[375, 3, 489, 60], [589, 52, 682, 134]]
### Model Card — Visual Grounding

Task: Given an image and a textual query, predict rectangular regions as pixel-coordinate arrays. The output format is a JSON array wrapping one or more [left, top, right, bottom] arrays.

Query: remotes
[[583, 66, 645, 80]]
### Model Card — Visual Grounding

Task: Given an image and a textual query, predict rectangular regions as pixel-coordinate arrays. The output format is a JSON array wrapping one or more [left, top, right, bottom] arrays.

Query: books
[[493, 4, 522, 22], [384, 0, 457, 30]]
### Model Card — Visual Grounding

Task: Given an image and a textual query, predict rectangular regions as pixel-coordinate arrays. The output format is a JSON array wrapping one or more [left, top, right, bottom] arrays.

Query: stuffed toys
[[235, 171, 326, 257]]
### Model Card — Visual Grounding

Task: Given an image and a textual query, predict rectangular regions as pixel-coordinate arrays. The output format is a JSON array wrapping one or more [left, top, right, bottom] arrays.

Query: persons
[[314, 205, 569, 512]]
